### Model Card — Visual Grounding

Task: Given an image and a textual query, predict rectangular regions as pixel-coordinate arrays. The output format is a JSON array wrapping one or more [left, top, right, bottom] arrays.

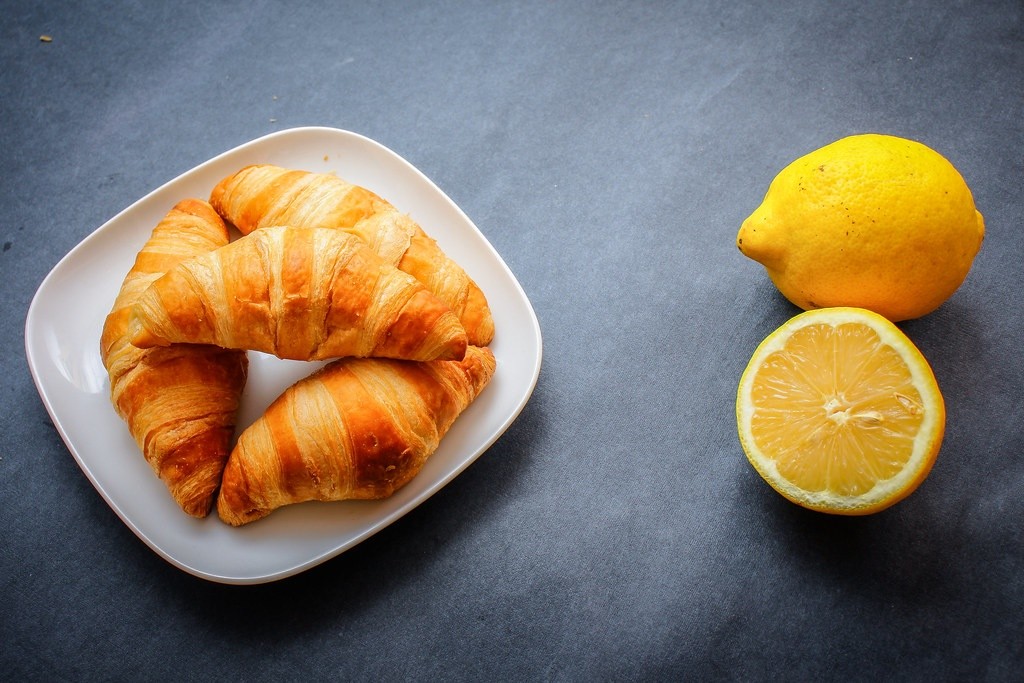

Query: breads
[[98, 199, 251, 520], [206, 163, 495, 349], [214, 342, 497, 527], [121, 226, 469, 363]]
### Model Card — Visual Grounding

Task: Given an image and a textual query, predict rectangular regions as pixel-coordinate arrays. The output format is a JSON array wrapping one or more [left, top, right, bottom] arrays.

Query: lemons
[[736, 305, 946, 515], [737, 134, 985, 322]]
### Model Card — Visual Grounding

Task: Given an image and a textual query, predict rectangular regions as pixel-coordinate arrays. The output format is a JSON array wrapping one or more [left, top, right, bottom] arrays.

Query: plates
[[23, 125, 543, 587]]
